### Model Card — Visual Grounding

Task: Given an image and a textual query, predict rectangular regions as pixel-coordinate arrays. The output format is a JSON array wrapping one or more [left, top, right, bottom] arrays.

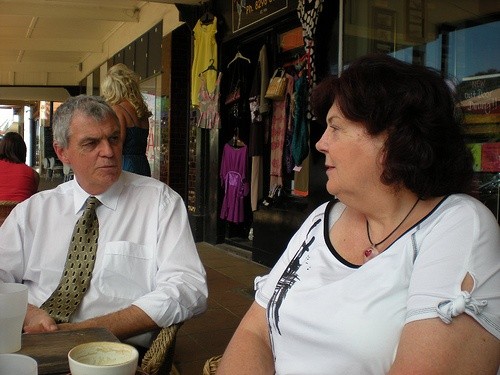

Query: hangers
[[194, 6, 219, 24], [224, 127, 248, 150], [198, 58, 224, 77], [226, 48, 250, 69]]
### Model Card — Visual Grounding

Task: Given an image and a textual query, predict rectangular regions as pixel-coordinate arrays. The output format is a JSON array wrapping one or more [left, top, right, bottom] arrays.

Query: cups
[[0, 283, 28, 354], [67, 342, 139, 375], [0, 354, 38, 375]]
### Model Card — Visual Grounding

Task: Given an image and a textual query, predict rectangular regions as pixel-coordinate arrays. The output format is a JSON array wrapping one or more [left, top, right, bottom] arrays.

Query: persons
[[214, 52, 500, 375], [0, 94, 210, 363], [1, 131, 40, 204], [99, 62, 152, 182]]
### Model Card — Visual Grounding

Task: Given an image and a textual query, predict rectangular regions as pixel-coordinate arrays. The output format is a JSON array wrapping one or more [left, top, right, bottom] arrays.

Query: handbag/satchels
[[264, 68, 288, 101]]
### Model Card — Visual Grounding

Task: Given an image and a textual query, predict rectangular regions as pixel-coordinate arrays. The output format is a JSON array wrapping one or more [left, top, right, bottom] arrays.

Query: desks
[[0, 326, 150, 375]]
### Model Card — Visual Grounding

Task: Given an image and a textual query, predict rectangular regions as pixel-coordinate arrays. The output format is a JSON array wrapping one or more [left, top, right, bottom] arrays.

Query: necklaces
[[363, 197, 422, 259]]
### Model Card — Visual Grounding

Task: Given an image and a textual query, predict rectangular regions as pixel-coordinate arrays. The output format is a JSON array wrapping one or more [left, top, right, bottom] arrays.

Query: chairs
[[49, 157, 63, 182], [43, 158, 51, 181], [203, 354, 224, 375], [136, 323, 183, 375], [63, 164, 74, 182], [0, 201, 21, 226]]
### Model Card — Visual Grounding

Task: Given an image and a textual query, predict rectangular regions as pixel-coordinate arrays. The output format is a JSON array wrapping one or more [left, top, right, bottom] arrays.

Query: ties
[[37, 196, 102, 325]]
